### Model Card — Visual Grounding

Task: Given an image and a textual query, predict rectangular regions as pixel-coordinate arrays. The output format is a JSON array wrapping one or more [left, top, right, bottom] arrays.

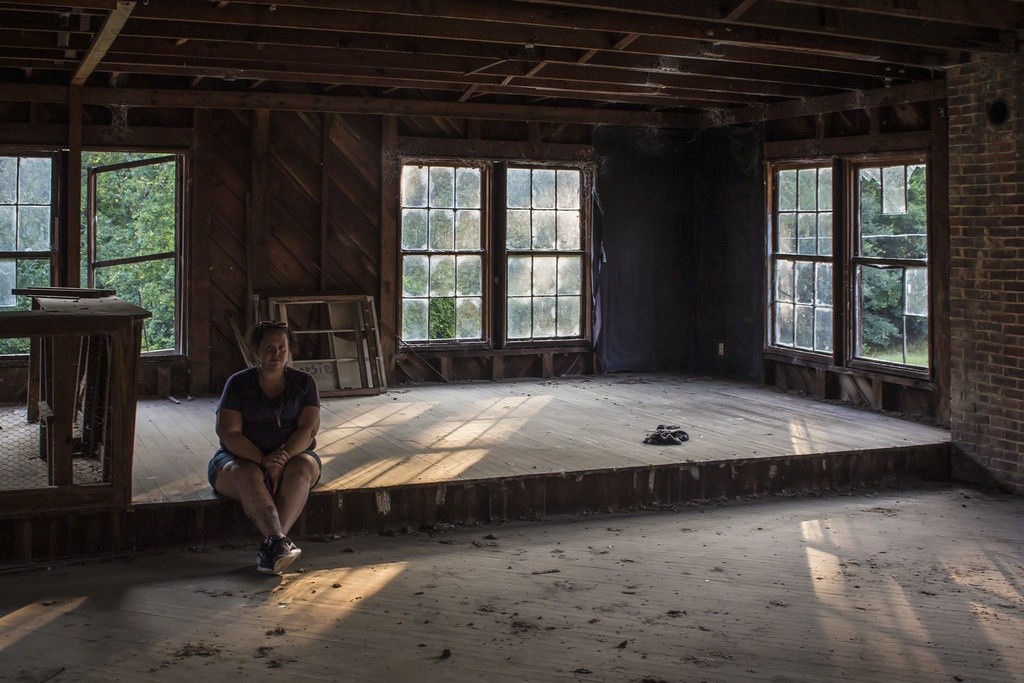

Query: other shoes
[[265, 534, 302, 573], [255, 542, 283, 577]]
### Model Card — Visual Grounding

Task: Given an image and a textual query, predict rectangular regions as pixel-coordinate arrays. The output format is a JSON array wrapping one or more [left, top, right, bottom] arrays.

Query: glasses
[[256, 319, 289, 342]]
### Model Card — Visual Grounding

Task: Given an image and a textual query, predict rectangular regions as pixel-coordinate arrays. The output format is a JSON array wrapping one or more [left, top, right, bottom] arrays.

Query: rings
[[272, 458, 277, 462]]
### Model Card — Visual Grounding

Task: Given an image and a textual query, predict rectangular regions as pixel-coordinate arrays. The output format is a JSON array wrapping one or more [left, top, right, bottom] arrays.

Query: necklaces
[[257, 366, 287, 428]]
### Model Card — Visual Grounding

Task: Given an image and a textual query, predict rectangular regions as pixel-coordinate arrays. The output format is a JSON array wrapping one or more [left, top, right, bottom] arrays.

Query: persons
[[208, 321, 323, 575]]
